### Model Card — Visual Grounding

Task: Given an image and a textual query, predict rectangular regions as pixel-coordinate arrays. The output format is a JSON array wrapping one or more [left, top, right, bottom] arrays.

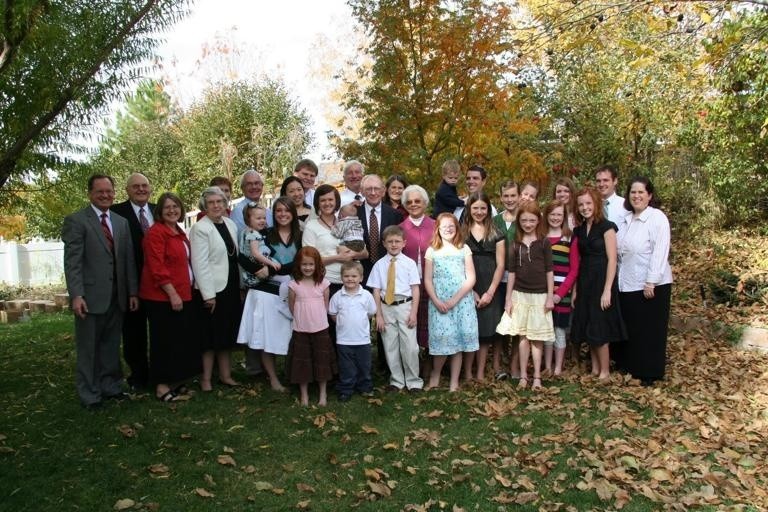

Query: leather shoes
[[87, 373, 242, 410]]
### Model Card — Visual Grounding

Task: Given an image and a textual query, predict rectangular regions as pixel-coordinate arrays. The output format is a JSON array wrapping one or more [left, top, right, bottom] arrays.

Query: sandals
[[510, 367, 610, 392]]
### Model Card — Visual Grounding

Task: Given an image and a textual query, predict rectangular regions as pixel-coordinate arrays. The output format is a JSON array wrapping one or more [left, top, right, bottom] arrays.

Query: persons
[[63, 158, 674, 409]]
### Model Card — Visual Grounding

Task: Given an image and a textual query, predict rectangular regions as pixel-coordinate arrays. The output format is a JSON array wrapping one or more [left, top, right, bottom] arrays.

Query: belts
[[380, 297, 411, 306]]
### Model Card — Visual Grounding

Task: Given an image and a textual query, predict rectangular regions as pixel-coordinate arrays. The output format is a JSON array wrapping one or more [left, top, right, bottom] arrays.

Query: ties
[[369, 209, 380, 264], [385, 257, 397, 305], [139, 207, 150, 233], [100, 213, 115, 252], [602, 200, 609, 220]]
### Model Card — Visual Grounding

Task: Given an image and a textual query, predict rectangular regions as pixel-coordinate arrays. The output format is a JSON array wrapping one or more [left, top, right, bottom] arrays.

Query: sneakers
[[299, 364, 508, 406]]
